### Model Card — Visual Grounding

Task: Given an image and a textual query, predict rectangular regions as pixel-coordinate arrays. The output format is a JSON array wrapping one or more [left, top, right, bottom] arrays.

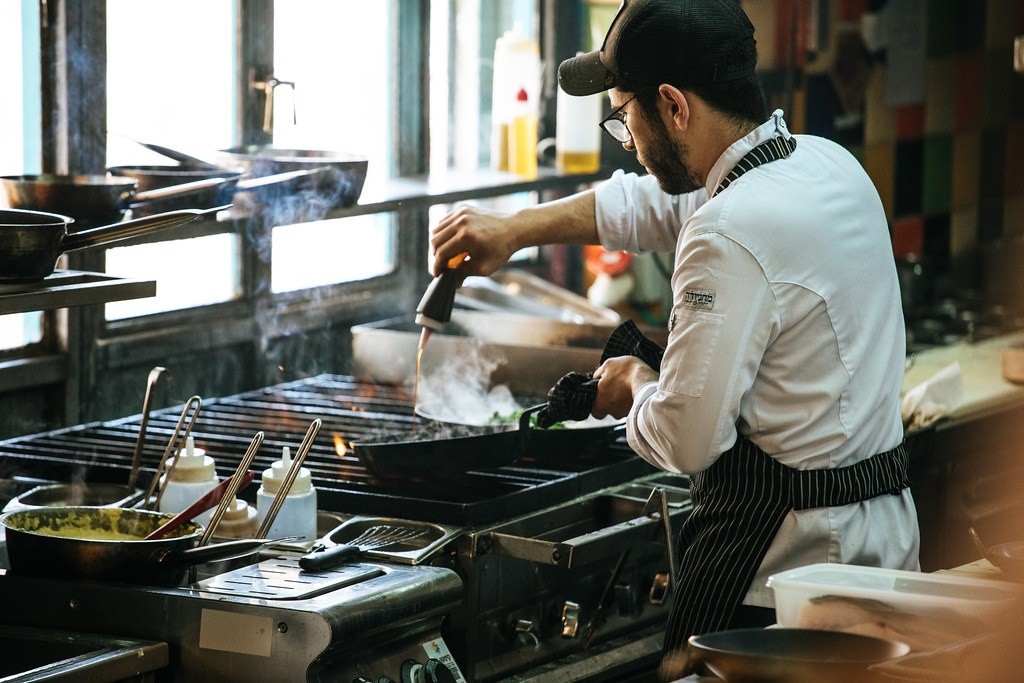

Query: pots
[[349, 376, 601, 467], [135, 139, 369, 209], [0, 202, 231, 284], [105, 165, 331, 222], [0, 506, 304, 627], [0, 172, 250, 230]]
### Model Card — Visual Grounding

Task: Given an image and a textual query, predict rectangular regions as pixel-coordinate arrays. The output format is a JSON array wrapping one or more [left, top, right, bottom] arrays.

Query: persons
[[429, 1, 920, 683]]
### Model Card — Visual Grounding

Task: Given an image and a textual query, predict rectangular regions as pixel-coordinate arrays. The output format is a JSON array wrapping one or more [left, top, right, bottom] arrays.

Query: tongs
[[578, 487, 680, 653]]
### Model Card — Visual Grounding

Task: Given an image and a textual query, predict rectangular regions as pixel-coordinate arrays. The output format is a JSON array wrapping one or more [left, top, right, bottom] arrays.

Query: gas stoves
[[0, 374, 691, 657]]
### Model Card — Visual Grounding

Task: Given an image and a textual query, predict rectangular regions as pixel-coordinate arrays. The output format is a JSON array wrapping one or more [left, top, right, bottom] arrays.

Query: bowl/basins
[[688, 629, 911, 683]]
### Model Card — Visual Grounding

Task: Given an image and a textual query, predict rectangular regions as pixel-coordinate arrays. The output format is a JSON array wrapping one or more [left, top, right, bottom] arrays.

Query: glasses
[[599, 95, 642, 142]]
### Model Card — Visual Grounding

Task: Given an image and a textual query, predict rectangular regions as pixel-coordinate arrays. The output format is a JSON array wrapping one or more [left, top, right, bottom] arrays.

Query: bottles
[[490, 21, 541, 173], [556, 55, 601, 172], [414, 244, 467, 349], [256, 448, 317, 545], [509, 89, 538, 181], [156, 437, 219, 530], [210, 490, 255, 539]]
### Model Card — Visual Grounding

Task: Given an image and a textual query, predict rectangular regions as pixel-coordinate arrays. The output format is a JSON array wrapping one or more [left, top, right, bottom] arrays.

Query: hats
[[558, 0, 758, 96]]
